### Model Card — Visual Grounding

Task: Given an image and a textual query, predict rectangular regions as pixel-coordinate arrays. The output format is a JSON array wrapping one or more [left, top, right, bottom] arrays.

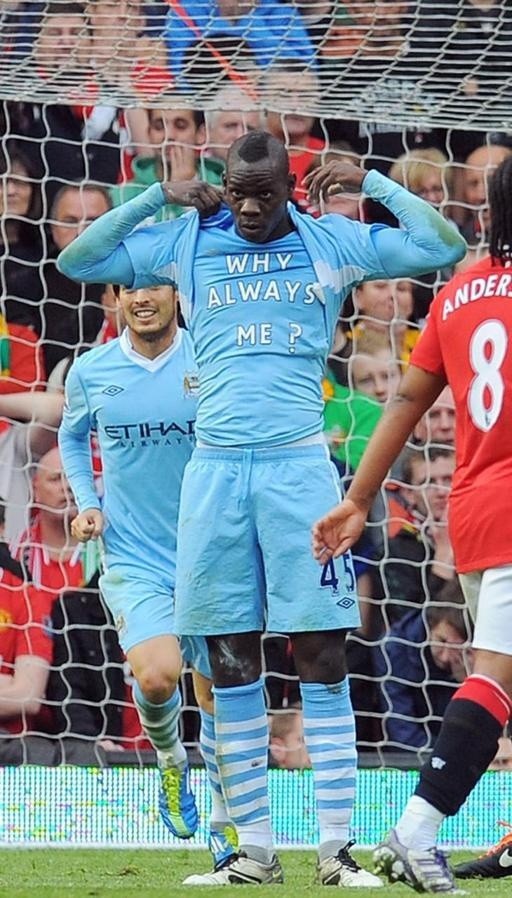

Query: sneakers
[[156, 752, 199, 839], [316, 839, 382, 889], [370, 830, 469, 896], [184, 829, 283, 885], [453, 820, 512, 878]]
